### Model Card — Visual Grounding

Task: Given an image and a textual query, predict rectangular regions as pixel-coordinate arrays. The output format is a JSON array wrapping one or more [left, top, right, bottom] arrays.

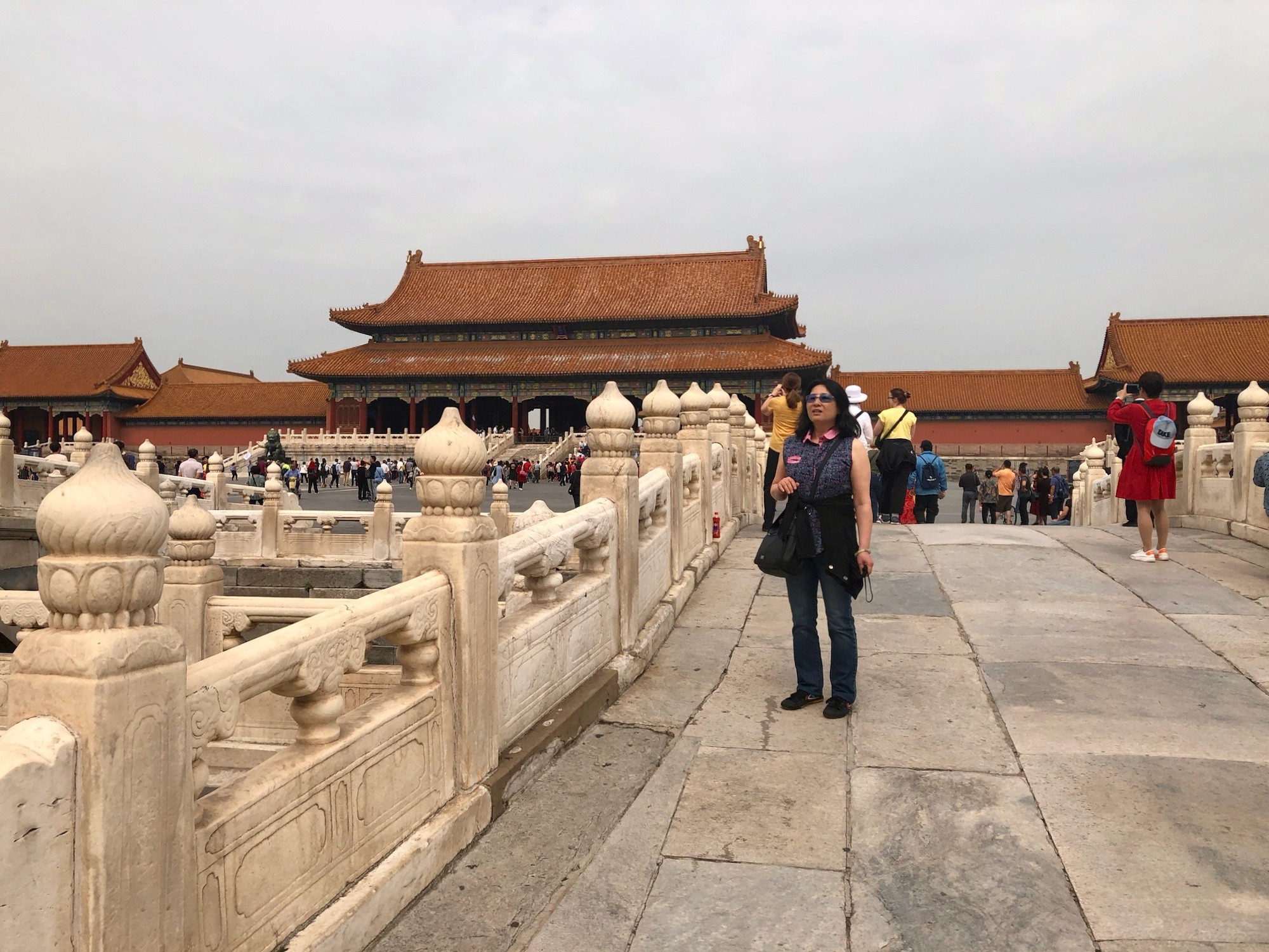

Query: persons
[[113, 441, 134, 470], [1253, 450, 1269, 518], [493, 425, 590, 442], [231, 456, 420, 506], [1107, 371, 1177, 561], [14, 441, 68, 483], [959, 459, 1074, 525], [481, 458, 541, 491], [158, 449, 210, 498], [545, 441, 590, 508], [770, 379, 873, 718], [761, 373, 947, 533]]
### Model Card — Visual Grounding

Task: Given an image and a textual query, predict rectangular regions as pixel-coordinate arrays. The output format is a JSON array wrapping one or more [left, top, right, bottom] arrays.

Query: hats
[[493, 461, 498, 466], [845, 385, 867, 403], [1150, 417, 1177, 449]]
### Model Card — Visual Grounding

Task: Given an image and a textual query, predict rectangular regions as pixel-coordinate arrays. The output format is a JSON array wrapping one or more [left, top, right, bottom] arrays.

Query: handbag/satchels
[[1030, 491, 1040, 515], [975, 489, 979, 500], [754, 509, 811, 578], [520, 471, 528, 476], [492, 467, 496, 476]]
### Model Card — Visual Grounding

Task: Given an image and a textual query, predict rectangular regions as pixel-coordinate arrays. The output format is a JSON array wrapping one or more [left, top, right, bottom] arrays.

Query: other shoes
[[518, 487, 523, 491], [877, 515, 900, 524], [319, 484, 359, 488], [357, 498, 376, 503], [509, 485, 516, 489], [761, 524, 775, 532], [547, 478, 566, 486], [1031, 522, 1038, 525], [1122, 520, 1140, 527]]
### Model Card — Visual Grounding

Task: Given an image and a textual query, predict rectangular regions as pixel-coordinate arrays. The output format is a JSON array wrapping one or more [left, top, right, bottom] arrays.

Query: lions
[[262, 431, 285, 458]]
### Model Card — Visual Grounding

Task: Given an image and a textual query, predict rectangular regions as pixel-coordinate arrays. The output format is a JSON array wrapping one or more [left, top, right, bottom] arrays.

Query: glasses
[[887, 396, 895, 399], [804, 394, 835, 403]]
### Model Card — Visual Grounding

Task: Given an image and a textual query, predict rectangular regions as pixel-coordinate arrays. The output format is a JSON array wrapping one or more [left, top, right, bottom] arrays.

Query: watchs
[[768, 393, 775, 397]]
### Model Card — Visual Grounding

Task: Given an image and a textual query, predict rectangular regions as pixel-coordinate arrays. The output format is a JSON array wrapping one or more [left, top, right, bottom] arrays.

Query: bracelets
[[1115, 396, 1123, 401], [854, 548, 871, 557]]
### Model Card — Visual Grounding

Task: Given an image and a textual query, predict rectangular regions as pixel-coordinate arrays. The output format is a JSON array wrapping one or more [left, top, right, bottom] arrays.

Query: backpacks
[[320, 463, 338, 476], [1144, 416, 1177, 467], [1057, 477, 1069, 498], [511, 464, 515, 469], [230, 466, 234, 471], [921, 462, 939, 489]]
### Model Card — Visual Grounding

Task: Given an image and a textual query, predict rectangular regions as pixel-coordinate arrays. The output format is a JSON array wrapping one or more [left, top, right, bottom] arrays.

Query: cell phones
[[1127, 384, 1139, 393]]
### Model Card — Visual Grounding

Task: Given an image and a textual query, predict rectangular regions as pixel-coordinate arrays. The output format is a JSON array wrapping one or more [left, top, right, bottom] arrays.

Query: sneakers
[[1131, 546, 1169, 562], [780, 691, 823, 709], [824, 697, 853, 719]]
